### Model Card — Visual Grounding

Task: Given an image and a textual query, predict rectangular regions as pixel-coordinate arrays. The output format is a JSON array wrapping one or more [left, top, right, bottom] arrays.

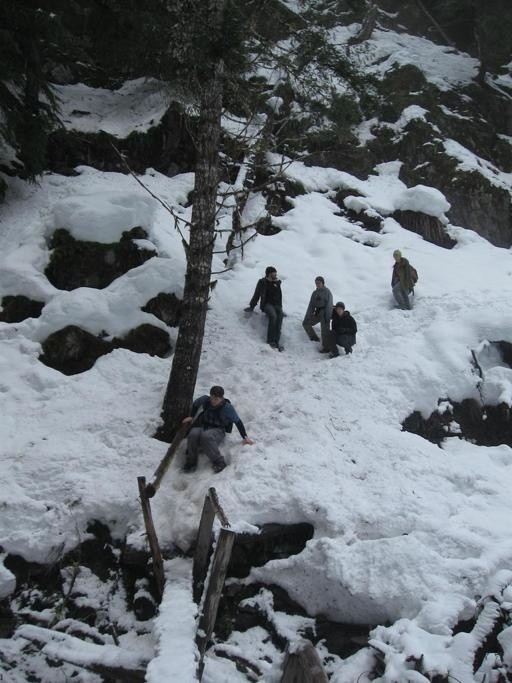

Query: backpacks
[[410, 266, 418, 283]]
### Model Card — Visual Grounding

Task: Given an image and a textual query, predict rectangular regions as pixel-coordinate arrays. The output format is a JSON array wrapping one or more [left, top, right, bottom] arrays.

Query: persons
[[329, 300, 358, 357], [180, 386, 254, 473], [243, 266, 289, 352], [390, 248, 415, 310], [302, 275, 334, 354]]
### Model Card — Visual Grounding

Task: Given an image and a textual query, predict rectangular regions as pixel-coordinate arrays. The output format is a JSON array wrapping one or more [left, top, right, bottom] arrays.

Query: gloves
[[335, 329, 344, 334], [244, 307, 253, 311]]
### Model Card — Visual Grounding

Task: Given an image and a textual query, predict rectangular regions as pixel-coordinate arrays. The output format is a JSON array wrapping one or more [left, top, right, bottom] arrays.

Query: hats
[[266, 267, 276, 275], [336, 302, 345, 311], [316, 276, 325, 286]]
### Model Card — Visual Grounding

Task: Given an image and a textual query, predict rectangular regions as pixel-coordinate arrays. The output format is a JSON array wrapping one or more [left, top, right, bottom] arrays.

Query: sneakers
[[182, 462, 197, 471], [213, 461, 226, 473]]
